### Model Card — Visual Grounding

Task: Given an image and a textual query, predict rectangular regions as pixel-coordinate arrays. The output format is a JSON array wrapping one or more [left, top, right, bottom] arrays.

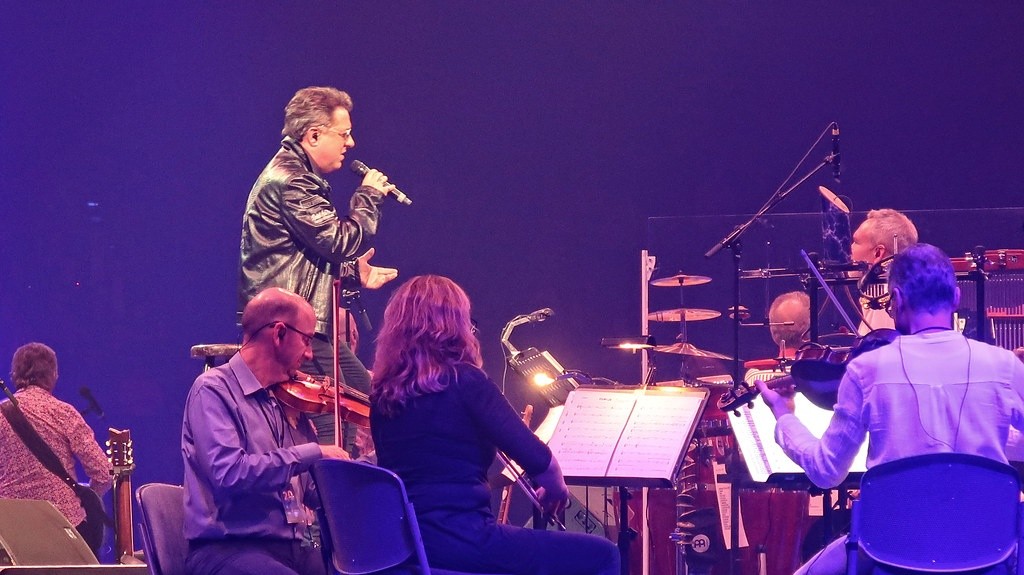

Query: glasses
[[270, 322, 314, 347], [885, 286, 904, 319], [470, 318, 478, 335], [323, 128, 352, 140]]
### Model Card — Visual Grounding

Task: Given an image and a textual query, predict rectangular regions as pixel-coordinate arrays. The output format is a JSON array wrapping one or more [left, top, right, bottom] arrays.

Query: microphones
[[350, 160, 413, 205], [830, 123, 841, 179]]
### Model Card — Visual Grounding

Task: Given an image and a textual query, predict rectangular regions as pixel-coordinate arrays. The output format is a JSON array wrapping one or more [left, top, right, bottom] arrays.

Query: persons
[[239, 84, 399, 453], [178, 288, 349, 575], [848, 209, 918, 337], [752, 243, 1024, 575], [744, 292, 811, 387], [0, 341, 110, 553], [369, 275, 622, 574]]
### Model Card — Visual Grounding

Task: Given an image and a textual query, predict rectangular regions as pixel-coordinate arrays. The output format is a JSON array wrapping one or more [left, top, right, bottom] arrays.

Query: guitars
[[73, 482, 107, 550], [105, 426, 137, 567]]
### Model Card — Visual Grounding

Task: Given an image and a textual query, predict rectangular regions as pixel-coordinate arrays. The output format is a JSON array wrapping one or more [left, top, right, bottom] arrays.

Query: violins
[[717, 327, 908, 417], [272, 368, 372, 430]]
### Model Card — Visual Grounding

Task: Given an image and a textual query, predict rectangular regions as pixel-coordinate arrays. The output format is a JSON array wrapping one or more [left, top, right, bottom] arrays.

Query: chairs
[[133, 483, 189, 575], [845, 451, 1024, 575], [305, 458, 483, 574]]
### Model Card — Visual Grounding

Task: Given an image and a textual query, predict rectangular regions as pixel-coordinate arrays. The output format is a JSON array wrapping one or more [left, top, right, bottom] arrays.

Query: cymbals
[[649, 341, 746, 362], [648, 307, 721, 322], [650, 274, 713, 286]]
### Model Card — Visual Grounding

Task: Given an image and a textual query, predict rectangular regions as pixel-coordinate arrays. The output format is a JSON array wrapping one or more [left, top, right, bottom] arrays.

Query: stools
[[189, 340, 245, 372]]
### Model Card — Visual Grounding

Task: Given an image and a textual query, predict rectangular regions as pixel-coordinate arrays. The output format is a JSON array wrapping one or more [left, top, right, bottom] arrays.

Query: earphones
[[312, 133, 318, 141]]
